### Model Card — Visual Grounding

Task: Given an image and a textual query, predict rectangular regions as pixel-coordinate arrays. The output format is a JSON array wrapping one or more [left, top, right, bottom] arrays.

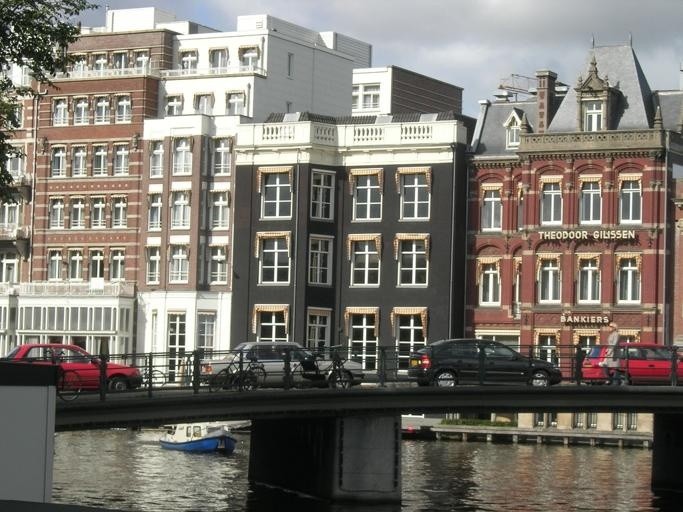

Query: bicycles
[[23, 350, 83, 402], [209, 349, 267, 393], [288, 347, 353, 389], [97, 352, 167, 391]]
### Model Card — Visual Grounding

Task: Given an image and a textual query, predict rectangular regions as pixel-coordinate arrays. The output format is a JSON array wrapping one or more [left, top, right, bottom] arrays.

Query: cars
[[580, 343, 682, 384], [199, 342, 365, 391], [6, 344, 143, 392], [407, 339, 562, 388]]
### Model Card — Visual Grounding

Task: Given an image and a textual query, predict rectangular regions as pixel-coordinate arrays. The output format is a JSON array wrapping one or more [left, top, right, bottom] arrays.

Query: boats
[[158, 424, 238, 454]]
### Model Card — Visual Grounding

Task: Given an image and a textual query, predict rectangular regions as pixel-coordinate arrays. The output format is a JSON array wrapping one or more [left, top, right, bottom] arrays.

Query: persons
[[604, 322, 626, 386]]
